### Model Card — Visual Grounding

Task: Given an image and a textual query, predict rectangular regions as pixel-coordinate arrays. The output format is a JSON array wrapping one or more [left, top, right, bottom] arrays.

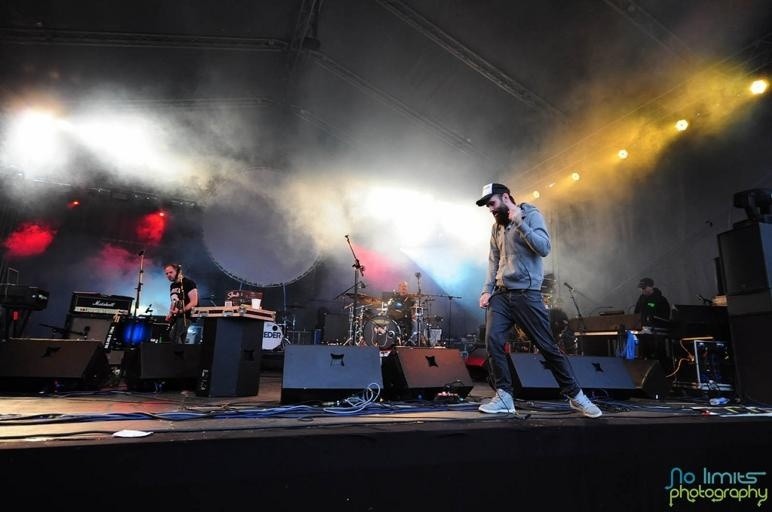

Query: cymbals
[[344, 293, 383, 304]]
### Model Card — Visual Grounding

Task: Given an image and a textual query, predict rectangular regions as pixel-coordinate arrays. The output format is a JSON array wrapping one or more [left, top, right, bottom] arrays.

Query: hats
[[637, 278, 654, 289], [476, 183, 510, 206]]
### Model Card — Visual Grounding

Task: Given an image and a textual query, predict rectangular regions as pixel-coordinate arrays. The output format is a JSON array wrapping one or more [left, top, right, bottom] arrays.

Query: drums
[[262, 321, 283, 351], [365, 315, 401, 350]]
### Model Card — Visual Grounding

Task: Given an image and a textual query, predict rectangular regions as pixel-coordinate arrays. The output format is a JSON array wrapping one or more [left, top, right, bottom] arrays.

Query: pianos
[[567, 314, 674, 337]]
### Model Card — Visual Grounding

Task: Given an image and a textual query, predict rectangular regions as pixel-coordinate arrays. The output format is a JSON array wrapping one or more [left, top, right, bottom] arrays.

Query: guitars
[[165, 300, 182, 321]]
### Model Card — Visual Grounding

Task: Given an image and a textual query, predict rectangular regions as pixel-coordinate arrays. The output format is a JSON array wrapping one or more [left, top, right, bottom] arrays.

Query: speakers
[[380, 347, 474, 402], [125, 341, 210, 394], [507, 353, 664, 401], [0, 336, 111, 394], [716, 220, 772, 406], [193, 314, 264, 398], [280, 343, 384, 405]]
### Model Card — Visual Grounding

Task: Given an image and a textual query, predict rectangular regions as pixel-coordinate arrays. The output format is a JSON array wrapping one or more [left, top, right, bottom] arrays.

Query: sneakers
[[569, 390, 602, 418], [478, 389, 515, 413]]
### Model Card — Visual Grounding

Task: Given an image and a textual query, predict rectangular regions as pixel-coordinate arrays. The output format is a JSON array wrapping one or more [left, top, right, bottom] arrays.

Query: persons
[[163, 263, 202, 398], [392, 282, 416, 340], [634, 278, 670, 359], [476, 183, 603, 419]]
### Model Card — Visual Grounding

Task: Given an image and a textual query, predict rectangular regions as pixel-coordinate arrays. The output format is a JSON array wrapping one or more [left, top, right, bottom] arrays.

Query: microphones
[[144, 303, 152, 313], [564, 282, 573, 289], [697, 294, 713, 305]]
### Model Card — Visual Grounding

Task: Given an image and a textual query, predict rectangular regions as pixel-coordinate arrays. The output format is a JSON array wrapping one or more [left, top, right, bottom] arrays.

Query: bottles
[[710, 398, 730, 405]]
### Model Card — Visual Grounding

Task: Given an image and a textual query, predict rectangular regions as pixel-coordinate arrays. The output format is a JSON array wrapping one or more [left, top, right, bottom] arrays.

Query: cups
[[252, 299, 261, 309]]
